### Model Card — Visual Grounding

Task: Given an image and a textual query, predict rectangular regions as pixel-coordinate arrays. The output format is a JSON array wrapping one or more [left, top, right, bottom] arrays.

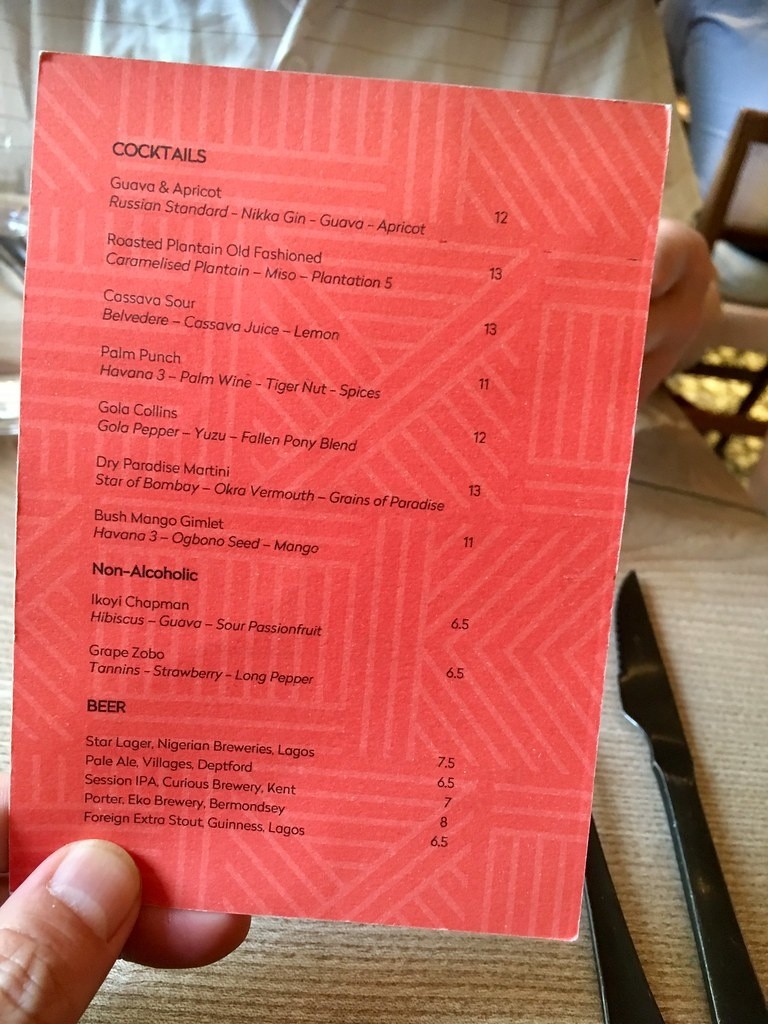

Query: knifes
[[614, 571, 767, 1022]]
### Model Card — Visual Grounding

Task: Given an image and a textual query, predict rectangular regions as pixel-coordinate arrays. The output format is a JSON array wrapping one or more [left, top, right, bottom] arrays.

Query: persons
[[1, 0, 730, 416]]
